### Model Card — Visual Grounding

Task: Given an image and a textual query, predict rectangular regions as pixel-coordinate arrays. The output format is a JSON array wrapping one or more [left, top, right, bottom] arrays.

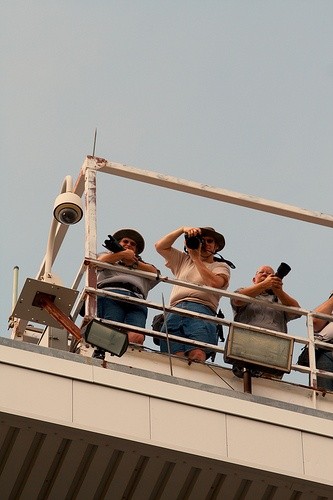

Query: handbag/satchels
[[297, 345, 309, 367], [151, 314, 164, 346]]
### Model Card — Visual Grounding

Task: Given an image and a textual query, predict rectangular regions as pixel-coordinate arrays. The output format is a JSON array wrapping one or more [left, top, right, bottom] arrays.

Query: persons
[[96, 229, 162, 349], [306, 290, 333, 392], [154, 225, 231, 365], [229, 265, 303, 380]]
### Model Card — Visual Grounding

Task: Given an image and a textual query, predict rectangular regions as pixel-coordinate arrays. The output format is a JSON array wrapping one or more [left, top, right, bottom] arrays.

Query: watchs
[[132, 261, 139, 269]]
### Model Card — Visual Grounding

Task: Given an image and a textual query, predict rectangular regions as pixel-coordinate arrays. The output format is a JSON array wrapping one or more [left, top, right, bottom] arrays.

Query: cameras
[[185, 234, 205, 250], [265, 263, 291, 302], [102, 235, 136, 266]]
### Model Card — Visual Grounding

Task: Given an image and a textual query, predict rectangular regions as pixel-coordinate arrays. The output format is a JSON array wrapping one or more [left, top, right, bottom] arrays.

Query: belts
[[98, 281, 143, 294]]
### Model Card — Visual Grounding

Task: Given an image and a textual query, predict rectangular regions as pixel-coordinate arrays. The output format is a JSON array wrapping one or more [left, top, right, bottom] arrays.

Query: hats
[[112, 229, 144, 255], [199, 227, 225, 252]]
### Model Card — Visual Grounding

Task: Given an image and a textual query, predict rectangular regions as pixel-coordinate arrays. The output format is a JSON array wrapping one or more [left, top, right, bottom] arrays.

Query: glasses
[[255, 271, 274, 275], [202, 239, 214, 243], [123, 240, 137, 246]]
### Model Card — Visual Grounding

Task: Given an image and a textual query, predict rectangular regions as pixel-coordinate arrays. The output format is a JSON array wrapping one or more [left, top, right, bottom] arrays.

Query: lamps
[[223, 325, 295, 374], [84, 319, 129, 358]]
